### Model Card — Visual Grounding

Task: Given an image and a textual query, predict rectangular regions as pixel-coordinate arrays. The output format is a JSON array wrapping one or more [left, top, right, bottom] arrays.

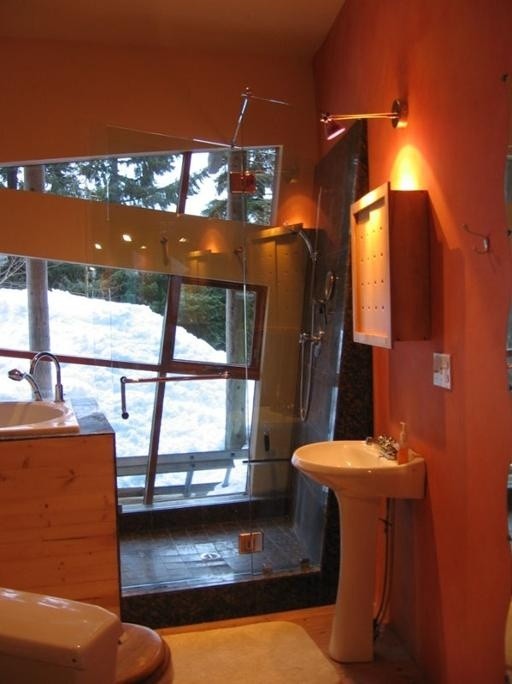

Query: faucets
[[366, 436, 387, 452], [29, 352, 61, 384]]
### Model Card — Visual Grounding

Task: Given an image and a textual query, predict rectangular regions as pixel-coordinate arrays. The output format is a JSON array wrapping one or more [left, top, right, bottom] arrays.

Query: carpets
[[159, 617, 343, 684]]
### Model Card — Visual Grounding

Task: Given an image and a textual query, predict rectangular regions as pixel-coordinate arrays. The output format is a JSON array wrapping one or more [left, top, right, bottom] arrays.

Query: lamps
[[319, 94, 409, 141]]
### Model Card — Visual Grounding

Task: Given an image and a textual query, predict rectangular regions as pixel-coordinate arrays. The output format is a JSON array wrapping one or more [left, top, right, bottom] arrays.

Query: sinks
[[291, 440, 425, 501], [0, 397, 80, 437]]
